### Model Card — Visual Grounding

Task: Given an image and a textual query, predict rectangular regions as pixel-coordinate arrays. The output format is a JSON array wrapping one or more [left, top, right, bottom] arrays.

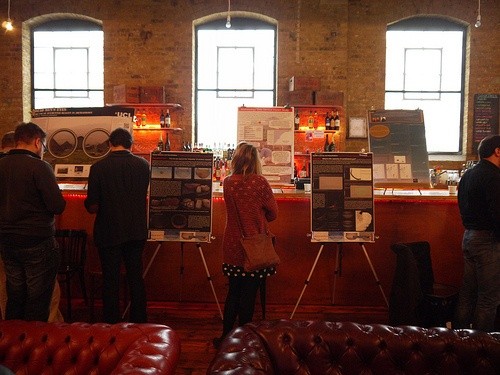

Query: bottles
[[158, 132, 163, 154], [329, 142, 335, 151], [160, 109, 165, 128], [182, 142, 213, 155], [335, 109, 340, 130], [324, 134, 329, 152], [326, 111, 330, 130], [330, 111, 335, 130], [305, 132, 314, 153], [165, 108, 171, 128], [213, 144, 238, 186], [165, 133, 170, 151], [308, 110, 319, 130], [291, 157, 311, 193], [136, 109, 141, 128], [294, 114, 300, 132], [141, 108, 146, 127]]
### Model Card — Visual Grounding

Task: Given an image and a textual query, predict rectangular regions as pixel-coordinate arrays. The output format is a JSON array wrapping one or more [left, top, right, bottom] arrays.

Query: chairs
[[390, 241, 460, 324], [56, 227, 91, 321]]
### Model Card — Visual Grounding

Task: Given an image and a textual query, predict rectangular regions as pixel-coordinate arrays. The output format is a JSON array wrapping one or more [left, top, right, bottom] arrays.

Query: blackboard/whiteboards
[[473, 93, 500, 154]]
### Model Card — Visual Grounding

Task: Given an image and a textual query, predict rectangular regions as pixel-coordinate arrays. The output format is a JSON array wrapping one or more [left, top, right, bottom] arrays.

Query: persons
[[458, 135, 500, 332], [84, 128, 152, 323], [0, 122, 66, 322], [214, 142, 277, 349]]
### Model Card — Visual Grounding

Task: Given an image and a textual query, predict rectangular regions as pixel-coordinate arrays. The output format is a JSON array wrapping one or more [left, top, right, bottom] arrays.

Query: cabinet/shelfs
[[108, 101, 184, 154], [292, 104, 348, 182]]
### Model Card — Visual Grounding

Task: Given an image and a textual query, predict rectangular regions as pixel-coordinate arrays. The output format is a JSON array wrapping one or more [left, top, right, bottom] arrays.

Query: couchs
[[206, 318, 500, 375], [0, 320, 182, 375]]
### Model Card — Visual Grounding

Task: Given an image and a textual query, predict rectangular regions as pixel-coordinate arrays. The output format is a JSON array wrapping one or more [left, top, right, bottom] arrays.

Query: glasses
[[39, 140, 48, 153]]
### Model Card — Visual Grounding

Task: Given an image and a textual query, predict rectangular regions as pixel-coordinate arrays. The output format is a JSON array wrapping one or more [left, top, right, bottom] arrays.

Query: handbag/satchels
[[240, 232, 281, 272]]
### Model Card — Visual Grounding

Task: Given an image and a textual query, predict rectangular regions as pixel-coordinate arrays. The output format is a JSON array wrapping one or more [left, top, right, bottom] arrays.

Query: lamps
[[2, 0, 14, 32], [226, 0, 232, 28], [475, 0, 481, 27]]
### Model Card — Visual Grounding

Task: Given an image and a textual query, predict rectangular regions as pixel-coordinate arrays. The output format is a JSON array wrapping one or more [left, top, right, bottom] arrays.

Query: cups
[[448, 185, 458, 194]]
